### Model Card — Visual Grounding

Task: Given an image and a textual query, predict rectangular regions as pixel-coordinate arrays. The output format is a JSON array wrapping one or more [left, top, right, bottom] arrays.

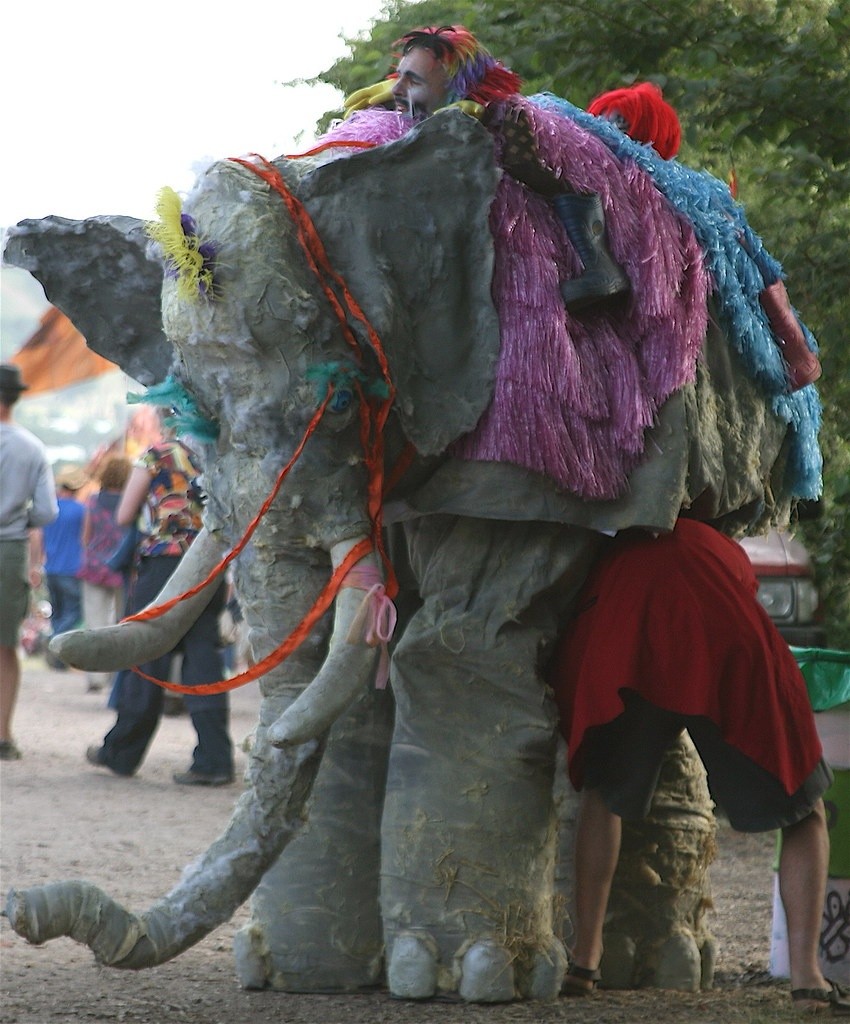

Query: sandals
[[560, 963, 602, 999], [791, 979, 850, 1017]]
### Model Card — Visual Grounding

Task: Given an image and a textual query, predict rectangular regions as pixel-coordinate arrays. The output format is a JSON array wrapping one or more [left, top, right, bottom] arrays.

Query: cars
[[737, 522, 830, 650]]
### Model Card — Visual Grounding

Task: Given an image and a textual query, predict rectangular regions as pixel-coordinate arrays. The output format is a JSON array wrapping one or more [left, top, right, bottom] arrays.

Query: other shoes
[[173, 769, 232, 785], [0, 740, 22, 760], [87, 746, 134, 777]]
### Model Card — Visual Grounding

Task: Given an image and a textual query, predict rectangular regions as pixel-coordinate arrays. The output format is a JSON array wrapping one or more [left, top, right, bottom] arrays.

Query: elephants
[[0, 101, 828, 1004]]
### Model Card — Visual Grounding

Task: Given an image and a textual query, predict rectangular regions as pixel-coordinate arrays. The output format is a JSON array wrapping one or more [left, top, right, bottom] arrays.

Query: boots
[[558, 192, 629, 311], [759, 279, 822, 392]]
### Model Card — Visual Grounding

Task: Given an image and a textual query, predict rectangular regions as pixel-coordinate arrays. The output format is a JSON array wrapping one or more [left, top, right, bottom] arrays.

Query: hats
[[57, 464, 88, 490], [0, 365, 29, 390]]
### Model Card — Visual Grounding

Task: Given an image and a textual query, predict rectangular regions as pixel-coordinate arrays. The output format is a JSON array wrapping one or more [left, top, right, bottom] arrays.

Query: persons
[[85, 401, 236, 786], [0, 360, 60, 760], [43, 459, 133, 694], [342, 23, 632, 313], [586, 80, 822, 390], [556, 517, 837, 997]]
